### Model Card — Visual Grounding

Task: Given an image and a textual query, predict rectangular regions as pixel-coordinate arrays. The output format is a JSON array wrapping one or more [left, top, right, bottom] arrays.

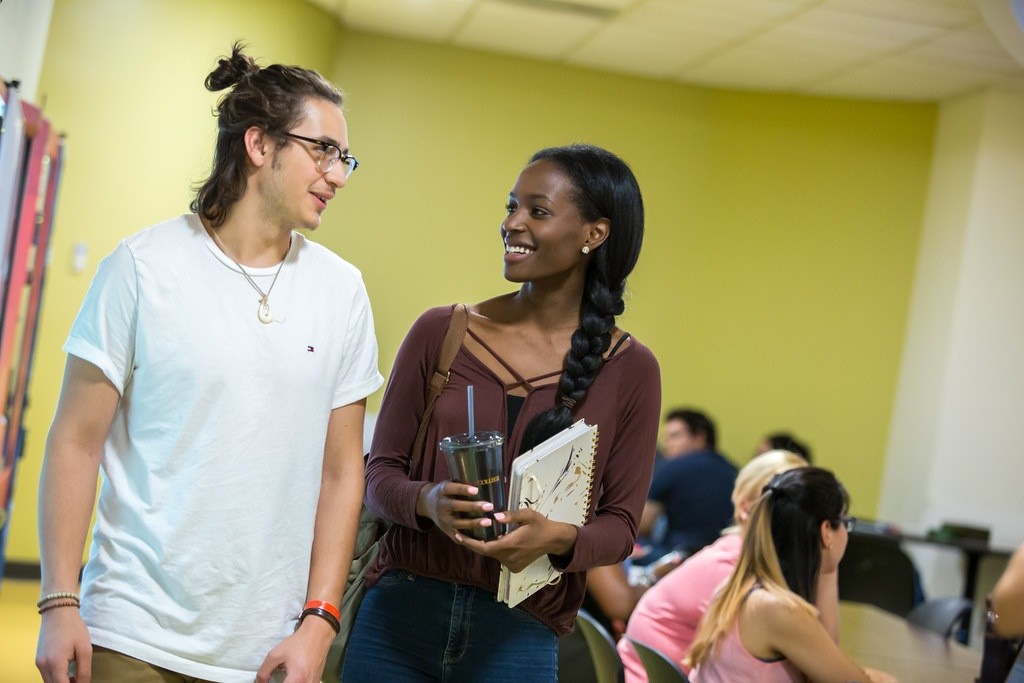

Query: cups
[[440, 432, 508, 542]]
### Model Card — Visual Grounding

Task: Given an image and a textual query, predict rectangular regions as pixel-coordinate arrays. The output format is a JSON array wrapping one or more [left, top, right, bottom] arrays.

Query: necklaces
[[205, 219, 289, 322]]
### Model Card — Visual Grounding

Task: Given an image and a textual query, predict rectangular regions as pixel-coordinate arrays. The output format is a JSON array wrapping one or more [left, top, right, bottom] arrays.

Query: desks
[[837, 600, 982, 683]]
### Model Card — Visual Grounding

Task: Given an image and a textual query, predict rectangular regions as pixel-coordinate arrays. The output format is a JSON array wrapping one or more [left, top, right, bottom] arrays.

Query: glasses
[[832, 516, 857, 534], [263, 124, 360, 179]]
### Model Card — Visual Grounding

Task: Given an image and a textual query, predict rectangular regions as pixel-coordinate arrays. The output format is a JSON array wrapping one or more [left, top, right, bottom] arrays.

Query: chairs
[[904, 600, 975, 636], [622, 632, 689, 683], [575, 607, 624, 683]]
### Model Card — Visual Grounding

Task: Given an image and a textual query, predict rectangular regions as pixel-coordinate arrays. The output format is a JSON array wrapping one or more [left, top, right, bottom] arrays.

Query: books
[[497, 419, 600, 609]]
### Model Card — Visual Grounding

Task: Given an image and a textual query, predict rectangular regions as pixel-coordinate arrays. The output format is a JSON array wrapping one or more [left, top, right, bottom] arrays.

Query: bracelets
[[37, 593, 80, 613], [637, 573, 657, 588], [299, 600, 341, 632]]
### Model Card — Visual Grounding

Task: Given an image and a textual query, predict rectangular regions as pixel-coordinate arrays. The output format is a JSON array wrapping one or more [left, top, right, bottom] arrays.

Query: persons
[[989, 542, 1023, 682], [36, 40, 384, 683], [687, 467, 872, 683], [615, 449, 896, 683], [581, 562, 680, 635], [749, 435, 810, 460], [341, 145, 662, 683], [626, 407, 738, 563]]
